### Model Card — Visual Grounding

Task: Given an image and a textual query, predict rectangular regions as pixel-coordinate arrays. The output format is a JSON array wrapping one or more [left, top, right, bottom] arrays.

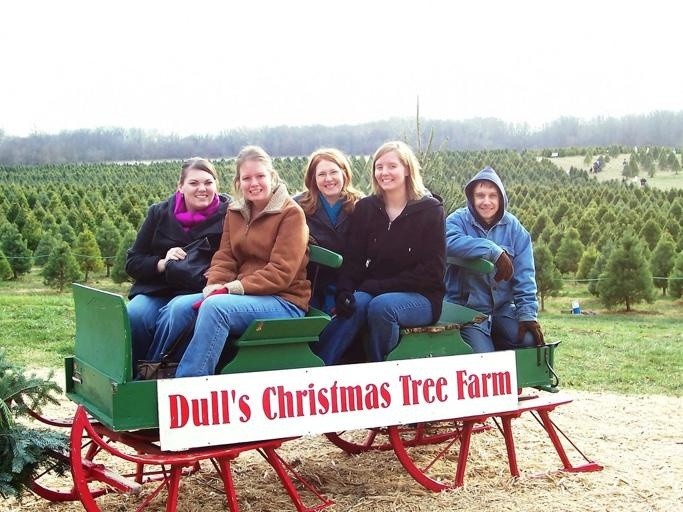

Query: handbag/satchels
[[166, 236, 214, 294], [134, 316, 197, 380]]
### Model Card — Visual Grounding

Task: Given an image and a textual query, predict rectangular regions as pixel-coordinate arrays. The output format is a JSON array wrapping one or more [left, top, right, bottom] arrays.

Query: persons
[[313, 140, 446, 366], [291, 148, 364, 315], [126, 158, 236, 381], [136, 145, 311, 377], [447, 165, 543, 353]]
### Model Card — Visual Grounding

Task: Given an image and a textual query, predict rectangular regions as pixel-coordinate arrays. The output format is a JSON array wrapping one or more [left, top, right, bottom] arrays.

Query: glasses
[[473, 192, 499, 199]]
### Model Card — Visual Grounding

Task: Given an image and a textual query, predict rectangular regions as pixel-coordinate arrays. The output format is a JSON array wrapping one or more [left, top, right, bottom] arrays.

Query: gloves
[[359, 279, 384, 294], [520, 320, 544, 347], [494, 251, 515, 282], [192, 286, 229, 310], [336, 289, 357, 319]]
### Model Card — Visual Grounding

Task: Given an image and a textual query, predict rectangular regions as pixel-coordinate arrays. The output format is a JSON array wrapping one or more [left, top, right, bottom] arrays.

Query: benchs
[[221, 244, 343, 375], [383, 257, 494, 361]]
